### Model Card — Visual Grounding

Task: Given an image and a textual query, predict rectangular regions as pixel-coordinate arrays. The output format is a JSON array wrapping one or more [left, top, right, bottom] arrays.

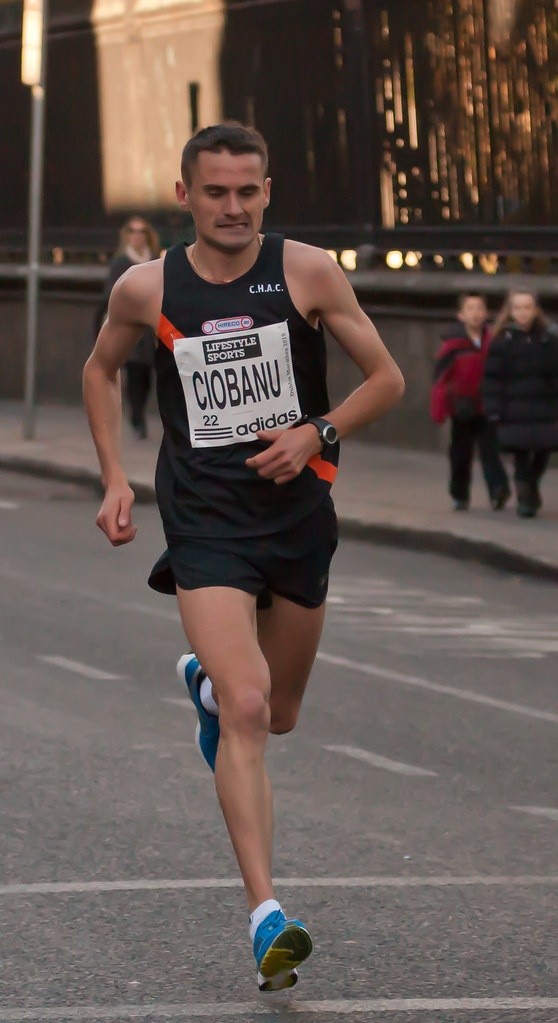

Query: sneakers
[[252, 909, 313, 993], [176, 652, 222, 774]]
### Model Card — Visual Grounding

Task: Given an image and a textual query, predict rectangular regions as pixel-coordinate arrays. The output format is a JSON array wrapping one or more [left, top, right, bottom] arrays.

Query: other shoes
[[519, 495, 543, 518], [456, 499, 470, 512], [493, 488, 510, 512]]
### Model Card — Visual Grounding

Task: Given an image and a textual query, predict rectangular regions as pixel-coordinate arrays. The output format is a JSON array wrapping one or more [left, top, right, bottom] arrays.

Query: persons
[[430, 291, 511, 510], [483, 289, 558, 519], [80, 121, 404, 992], [94, 217, 162, 436]]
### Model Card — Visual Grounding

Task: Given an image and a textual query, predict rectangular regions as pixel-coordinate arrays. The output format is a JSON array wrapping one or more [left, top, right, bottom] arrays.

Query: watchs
[[306, 417, 338, 449]]
